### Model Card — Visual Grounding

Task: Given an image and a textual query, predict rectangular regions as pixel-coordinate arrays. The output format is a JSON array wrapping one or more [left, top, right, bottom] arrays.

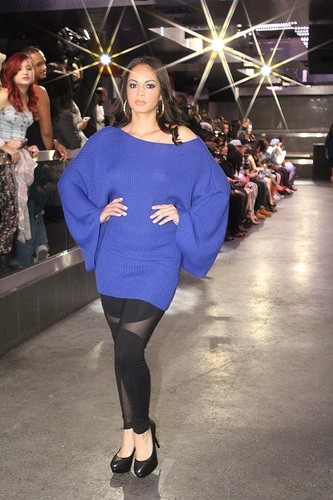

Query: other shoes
[[36, 245, 47, 261], [225, 186, 297, 240]]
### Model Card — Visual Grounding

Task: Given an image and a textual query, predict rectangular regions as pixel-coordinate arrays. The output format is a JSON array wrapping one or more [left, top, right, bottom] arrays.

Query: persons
[[57, 57, 232, 477], [173, 91, 298, 241], [0, 45, 128, 270]]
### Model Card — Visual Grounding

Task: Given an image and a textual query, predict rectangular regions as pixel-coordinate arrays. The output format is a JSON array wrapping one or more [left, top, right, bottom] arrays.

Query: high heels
[[134, 418, 160, 477], [111, 446, 135, 473]]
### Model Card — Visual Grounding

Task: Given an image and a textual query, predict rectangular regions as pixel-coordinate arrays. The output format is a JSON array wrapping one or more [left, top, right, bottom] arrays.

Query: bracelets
[[54, 139, 60, 143]]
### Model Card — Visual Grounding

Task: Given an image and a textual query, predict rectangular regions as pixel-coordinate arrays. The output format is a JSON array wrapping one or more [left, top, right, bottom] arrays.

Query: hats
[[230, 139, 243, 146], [200, 122, 216, 136]]
